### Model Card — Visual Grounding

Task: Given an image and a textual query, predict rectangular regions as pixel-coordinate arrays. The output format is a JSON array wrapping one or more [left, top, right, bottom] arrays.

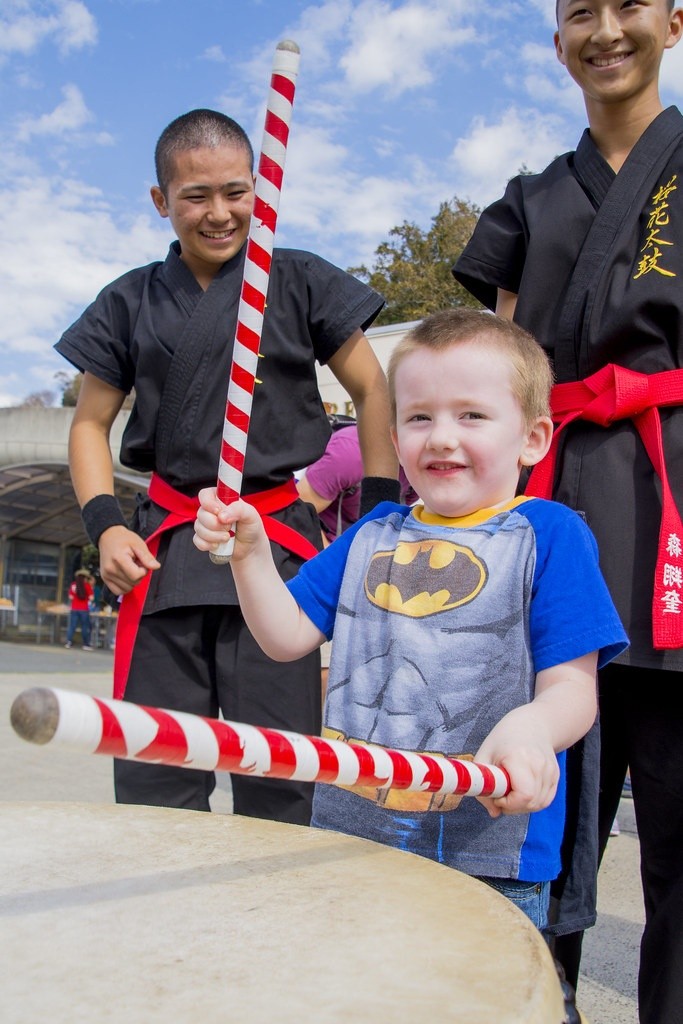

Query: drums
[[0, 801, 566, 1023]]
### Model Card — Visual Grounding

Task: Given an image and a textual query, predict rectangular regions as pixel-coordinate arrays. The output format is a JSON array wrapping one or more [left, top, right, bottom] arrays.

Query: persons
[[67, 568, 95, 651], [186, 310, 630, 929], [447, 0, 683, 1022], [296, 413, 366, 546], [54, 108, 394, 828]]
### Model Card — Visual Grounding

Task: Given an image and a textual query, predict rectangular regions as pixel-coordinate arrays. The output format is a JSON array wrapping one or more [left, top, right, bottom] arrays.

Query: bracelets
[[82, 495, 128, 546]]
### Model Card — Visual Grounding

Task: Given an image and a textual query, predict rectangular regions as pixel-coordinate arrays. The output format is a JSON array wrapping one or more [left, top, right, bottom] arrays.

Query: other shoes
[[83, 645, 93, 650], [621, 777, 634, 798], [65, 643, 70, 649], [608, 818, 621, 835]]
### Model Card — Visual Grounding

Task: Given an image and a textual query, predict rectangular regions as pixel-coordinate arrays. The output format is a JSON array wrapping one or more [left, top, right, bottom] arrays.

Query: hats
[[74, 568, 90, 578]]
